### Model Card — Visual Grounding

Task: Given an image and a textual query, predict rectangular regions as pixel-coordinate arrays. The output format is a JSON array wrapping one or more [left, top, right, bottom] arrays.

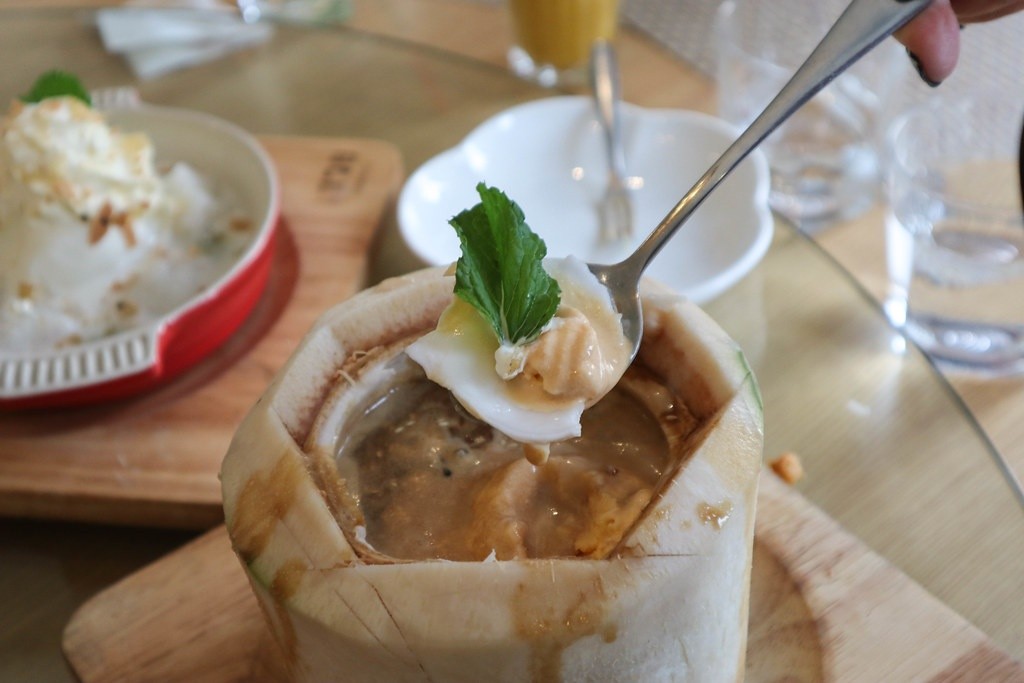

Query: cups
[[710, 0, 885, 224], [879, 47, 1024, 376], [502, 0, 621, 91]]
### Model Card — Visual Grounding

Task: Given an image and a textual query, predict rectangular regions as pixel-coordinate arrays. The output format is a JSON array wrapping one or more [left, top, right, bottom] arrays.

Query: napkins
[[94, 5, 271, 78]]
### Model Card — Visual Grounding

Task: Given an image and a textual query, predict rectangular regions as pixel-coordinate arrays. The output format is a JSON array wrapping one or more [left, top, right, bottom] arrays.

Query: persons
[[879, 0, 1024, 89]]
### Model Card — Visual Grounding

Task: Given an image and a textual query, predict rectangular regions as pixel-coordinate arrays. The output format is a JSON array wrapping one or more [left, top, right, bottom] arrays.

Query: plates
[[396, 95, 773, 305], [397, 89, 775, 303]]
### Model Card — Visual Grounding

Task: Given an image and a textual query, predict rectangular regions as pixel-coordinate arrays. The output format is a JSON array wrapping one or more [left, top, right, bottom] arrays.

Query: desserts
[[0, 99, 217, 352]]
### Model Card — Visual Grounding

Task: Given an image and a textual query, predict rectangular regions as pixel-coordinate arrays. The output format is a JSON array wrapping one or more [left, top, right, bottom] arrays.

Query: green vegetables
[[449, 182, 561, 349], [22, 68, 96, 104]]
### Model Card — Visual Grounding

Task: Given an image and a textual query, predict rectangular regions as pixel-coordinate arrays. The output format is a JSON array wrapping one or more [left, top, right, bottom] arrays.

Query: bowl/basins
[[0, 82, 280, 412]]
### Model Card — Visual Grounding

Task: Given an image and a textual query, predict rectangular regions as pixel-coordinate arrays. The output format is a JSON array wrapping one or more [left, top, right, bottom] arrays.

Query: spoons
[[434, 0, 957, 413]]
